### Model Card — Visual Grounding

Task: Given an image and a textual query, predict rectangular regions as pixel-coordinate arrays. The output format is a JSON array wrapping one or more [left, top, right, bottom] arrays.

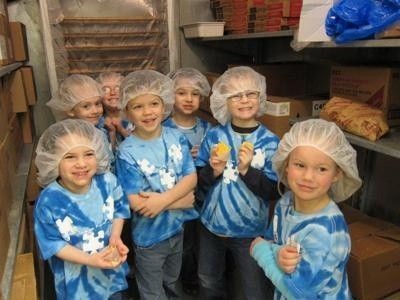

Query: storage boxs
[[0, 0, 42, 300]]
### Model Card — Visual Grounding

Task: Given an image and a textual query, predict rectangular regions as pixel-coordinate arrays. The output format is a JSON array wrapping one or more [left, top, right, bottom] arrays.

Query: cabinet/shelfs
[[192, 29, 400, 159]]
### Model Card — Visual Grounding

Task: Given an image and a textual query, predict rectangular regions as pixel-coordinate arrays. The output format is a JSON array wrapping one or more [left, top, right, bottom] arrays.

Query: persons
[[114, 71, 199, 300], [95, 70, 131, 152], [249, 118, 363, 300], [46, 72, 136, 280], [27, 119, 134, 300], [158, 67, 217, 296], [192, 64, 289, 300]]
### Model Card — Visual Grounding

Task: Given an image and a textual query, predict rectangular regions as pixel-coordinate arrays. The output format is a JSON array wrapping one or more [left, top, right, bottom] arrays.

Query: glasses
[[230, 91, 260, 101]]
